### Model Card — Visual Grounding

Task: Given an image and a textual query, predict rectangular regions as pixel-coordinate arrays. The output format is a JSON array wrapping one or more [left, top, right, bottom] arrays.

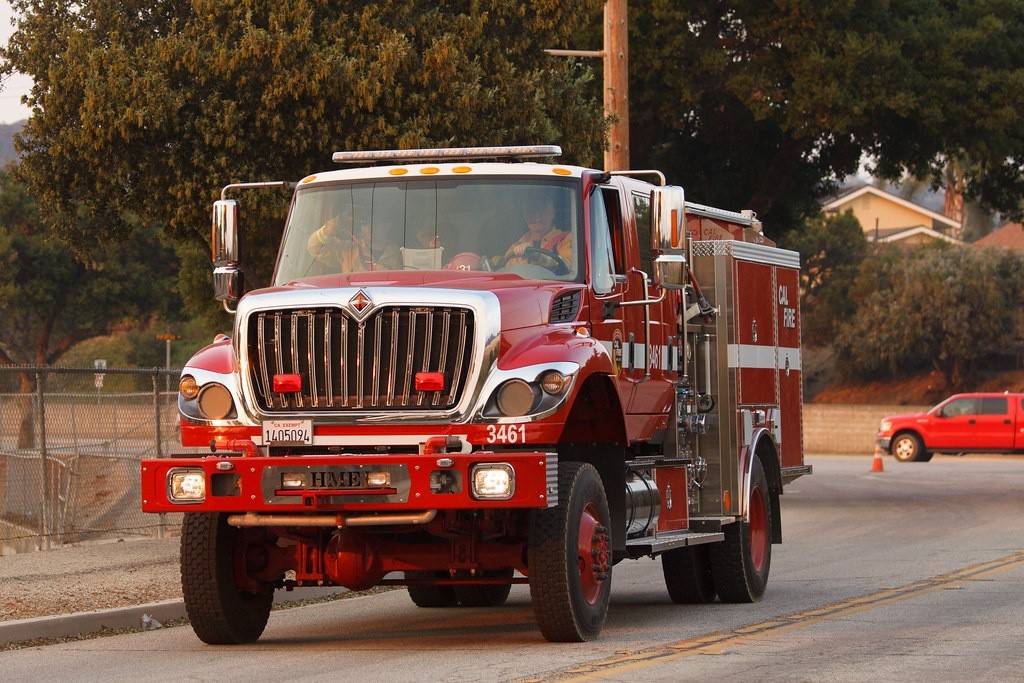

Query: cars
[[876, 390, 1024, 463]]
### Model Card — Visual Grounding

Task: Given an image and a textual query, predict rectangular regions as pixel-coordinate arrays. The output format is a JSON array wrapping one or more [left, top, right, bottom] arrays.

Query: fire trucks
[[141, 144, 813, 644]]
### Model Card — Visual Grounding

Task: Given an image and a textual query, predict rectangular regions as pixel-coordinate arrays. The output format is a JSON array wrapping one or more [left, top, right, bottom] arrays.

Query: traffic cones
[[871, 439, 886, 472]]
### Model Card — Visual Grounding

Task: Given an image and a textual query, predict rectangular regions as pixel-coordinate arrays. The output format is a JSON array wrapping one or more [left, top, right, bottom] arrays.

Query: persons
[[308, 209, 402, 273], [503, 198, 572, 269]]
